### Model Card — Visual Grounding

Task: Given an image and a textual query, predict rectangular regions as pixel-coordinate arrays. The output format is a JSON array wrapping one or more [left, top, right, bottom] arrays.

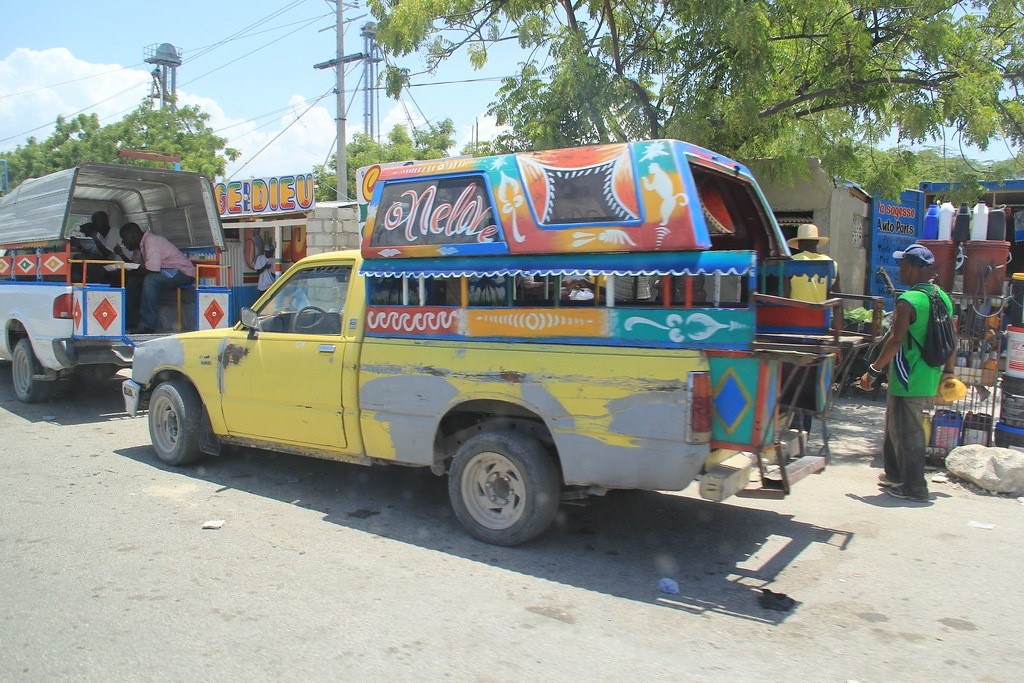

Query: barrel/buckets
[[932, 409, 962, 450], [1000, 372, 1024, 429], [915, 240, 956, 292], [995, 422, 1024, 449], [960, 411, 992, 447], [962, 240, 1011, 295], [1005, 325, 1024, 378], [1011, 273, 1024, 328]]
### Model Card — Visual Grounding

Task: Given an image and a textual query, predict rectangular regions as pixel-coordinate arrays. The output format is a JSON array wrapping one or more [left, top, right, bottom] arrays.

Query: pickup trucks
[[121, 137, 884, 547], [0, 161, 232, 402]]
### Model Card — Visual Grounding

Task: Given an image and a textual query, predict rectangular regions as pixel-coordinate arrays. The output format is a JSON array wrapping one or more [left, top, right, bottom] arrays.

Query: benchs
[[170, 246, 216, 333]]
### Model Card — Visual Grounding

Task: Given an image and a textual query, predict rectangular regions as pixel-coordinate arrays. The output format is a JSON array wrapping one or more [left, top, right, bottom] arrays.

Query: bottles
[[923, 205, 939, 239], [921, 412, 931, 447], [939, 202, 954, 241], [971, 201, 988, 239], [188, 252, 215, 260], [953, 203, 971, 241], [988, 205, 1006, 241]]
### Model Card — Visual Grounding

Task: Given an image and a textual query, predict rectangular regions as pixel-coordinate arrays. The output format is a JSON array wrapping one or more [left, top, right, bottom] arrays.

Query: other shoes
[[878, 473, 903, 487], [888, 485, 929, 502]]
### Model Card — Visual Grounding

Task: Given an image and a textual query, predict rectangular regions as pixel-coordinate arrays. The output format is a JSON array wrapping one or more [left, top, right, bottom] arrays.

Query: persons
[[860, 244, 958, 503], [255, 244, 275, 296], [114, 222, 196, 334], [786, 224, 844, 440], [70, 211, 133, 288]]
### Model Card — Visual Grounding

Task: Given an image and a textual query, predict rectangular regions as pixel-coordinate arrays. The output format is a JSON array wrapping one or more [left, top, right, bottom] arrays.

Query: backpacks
[[908, 284, 957, 367]]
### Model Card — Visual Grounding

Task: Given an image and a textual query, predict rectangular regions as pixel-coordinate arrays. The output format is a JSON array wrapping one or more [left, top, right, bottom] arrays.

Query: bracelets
[[867, 364, 883, 379], [943, 369, 953, 374]]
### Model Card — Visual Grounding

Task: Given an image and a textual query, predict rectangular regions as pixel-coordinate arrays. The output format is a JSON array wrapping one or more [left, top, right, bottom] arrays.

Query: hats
[[892, 244, 935, 265], [264, 244, 274, 251], [787, 224, 829, 249]]
